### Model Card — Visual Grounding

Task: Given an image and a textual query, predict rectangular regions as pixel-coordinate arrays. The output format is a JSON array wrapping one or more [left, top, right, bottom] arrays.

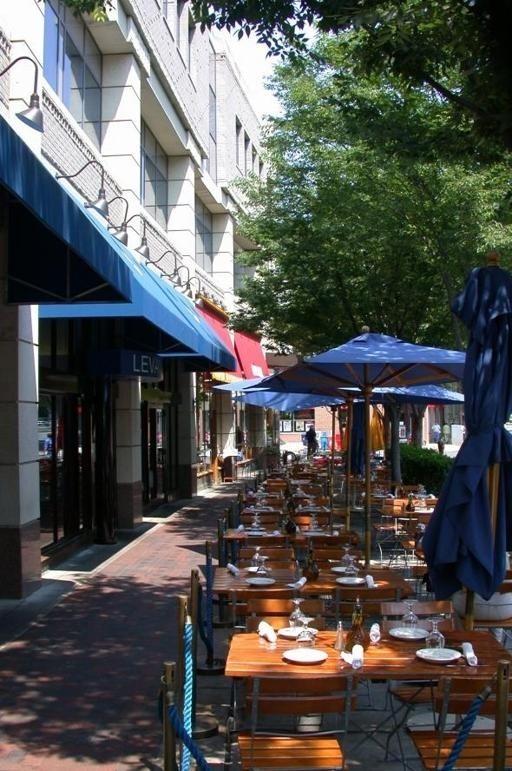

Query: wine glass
[[340, 545, 353, 570], [288, 598, 305, 633], [424, 617, 445, 656], [400, 600, 419, 635], [251, 513, 318, 532], [343, 556, 358, 583], [254, 495, 315, 510], [295, 617, 317, 657], [256, 557, 268, 583], [250, 547, 262, 568]]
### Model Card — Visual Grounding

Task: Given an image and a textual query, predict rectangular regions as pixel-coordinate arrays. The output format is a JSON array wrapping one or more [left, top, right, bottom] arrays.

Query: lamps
[[55, 159, 110, 216], [145, 250, 181, 286], [173, 276, 203, 308], [160, 265, 194, 299], [107, 215, 150, 260], [84, 197, 129, 246], [1, 56, 45, 133]]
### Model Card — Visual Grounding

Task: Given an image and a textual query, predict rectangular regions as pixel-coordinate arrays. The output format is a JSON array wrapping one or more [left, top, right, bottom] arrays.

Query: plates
[[246, 578, 274, 586], [330, 567, 360, 575], [336, 579, 366, 586], [278, 627, 318, 640], [416, 647, 461, 665], [389, 627, 429, 642], [283, 649, 328, 664], [246, 567, 271, 574], [244, 528, 323, 536]]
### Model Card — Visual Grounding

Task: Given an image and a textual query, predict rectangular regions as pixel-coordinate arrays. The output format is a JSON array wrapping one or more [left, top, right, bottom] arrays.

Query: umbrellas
[[228, 391, 399, 476], [211, 372, 465, 532], [243, 324, 466, 568], [421, 250, 511, 632]]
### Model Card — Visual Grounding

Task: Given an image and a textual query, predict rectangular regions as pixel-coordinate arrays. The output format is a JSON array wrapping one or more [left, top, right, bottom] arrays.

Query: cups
[[417, 489, 426, 511]]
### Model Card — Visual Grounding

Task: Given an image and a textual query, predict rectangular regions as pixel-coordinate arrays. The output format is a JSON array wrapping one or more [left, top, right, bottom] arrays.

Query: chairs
[[212, 457, 512, 771]]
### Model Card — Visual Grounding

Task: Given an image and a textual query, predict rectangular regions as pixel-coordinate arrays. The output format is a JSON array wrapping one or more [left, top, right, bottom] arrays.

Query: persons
[[448, 552, 512, 642], [42, 432, 53, 456], [462, 425, 466, 441], [432, 421, 441, 443], [305, 423, 318, 459], [443, 422, 450, 444]]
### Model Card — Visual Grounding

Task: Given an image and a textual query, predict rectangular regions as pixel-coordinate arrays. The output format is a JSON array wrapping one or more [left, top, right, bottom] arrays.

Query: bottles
[[304, 550, 319, 582], [293, 561, 300, 579], [335, 621, 345, 653], [406, 491, 415, 512], [345, 606, 368, 653]]
